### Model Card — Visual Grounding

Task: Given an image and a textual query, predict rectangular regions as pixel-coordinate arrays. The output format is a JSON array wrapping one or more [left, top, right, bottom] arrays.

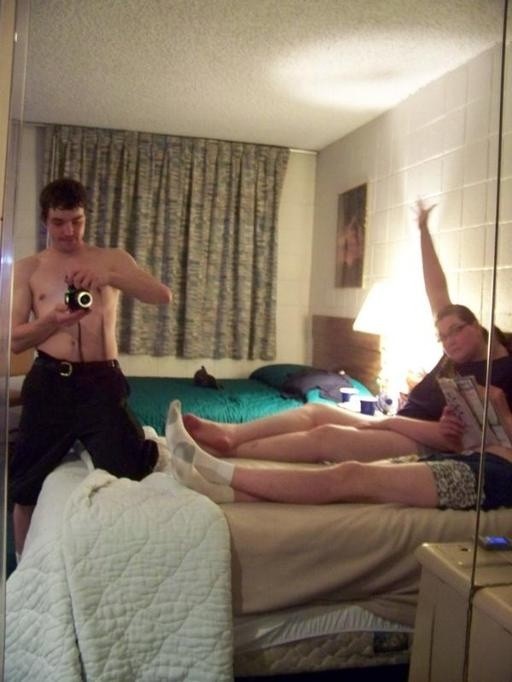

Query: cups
[[339, 387, 358, 402], [360, 399, 377, 416]]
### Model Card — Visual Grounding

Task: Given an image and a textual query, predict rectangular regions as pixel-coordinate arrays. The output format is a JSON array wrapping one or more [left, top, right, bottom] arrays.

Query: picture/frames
[[334, 179, 371, 289]]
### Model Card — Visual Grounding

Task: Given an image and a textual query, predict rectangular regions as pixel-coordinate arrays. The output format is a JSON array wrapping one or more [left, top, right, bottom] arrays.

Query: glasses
[[440, 323, 468, 342]]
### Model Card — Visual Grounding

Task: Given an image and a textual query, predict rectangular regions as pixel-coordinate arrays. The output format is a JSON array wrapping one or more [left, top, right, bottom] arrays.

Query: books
[[437, 373, 512, 450]]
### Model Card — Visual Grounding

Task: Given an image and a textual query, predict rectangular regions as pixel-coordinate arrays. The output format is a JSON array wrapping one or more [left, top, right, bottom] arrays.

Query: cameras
[[65, 283, 94, 310]]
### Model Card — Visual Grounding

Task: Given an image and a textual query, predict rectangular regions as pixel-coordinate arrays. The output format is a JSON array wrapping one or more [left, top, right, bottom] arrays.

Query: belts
[[34, 357, 116, 377]]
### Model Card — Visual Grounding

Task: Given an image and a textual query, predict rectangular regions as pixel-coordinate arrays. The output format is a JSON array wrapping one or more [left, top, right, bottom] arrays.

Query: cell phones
[[472, 535, 512, 550]]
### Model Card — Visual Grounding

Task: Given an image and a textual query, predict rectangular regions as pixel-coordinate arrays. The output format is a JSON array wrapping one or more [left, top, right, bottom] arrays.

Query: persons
[[164, 385, 512, 514], [5, 177, 173, 566], [182, 192, 512, 464]]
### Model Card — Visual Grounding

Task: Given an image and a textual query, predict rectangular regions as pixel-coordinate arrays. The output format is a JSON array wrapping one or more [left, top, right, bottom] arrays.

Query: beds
[[120, 314, 385, 436], [4, 429, 512, 681]]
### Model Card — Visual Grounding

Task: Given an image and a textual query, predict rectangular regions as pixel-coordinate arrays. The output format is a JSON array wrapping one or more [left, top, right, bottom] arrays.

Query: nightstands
[[407, 542, 512, 681]]
